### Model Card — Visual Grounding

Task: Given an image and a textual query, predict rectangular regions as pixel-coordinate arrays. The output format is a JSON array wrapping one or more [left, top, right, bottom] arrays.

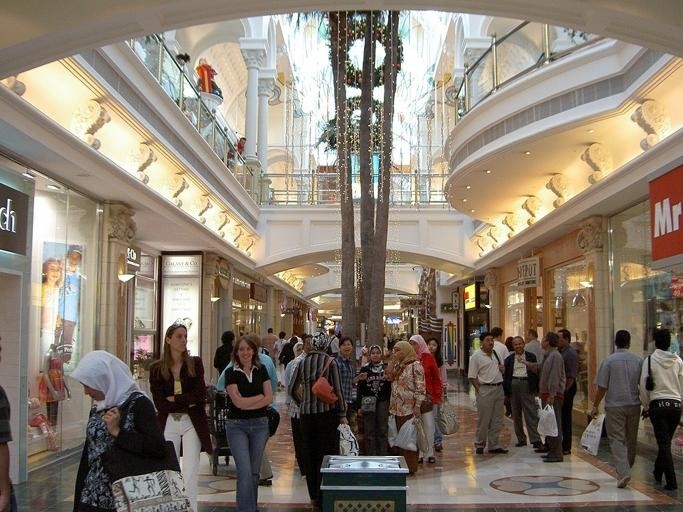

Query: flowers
[[133, 348, 152, 366], [311, 96, 397, 158], [327, 20, 403, 90]]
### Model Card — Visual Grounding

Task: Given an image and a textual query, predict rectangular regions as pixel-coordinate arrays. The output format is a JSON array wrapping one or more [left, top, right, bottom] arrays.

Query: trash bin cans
[[319, 455, 410, 512]]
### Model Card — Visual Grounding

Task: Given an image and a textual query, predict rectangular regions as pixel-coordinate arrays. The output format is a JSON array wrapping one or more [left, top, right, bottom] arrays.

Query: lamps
[[553, 268, 565, 311], [569, 264, 587, 308]]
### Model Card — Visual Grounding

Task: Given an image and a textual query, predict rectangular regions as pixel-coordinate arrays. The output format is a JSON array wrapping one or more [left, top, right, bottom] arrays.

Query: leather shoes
[[476, 448, 509, 454], [515, 443, 562, 462]]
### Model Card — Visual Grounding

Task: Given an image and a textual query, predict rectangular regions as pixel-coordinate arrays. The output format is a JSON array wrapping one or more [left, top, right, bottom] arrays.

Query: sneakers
[[617, 474, 630, 488]]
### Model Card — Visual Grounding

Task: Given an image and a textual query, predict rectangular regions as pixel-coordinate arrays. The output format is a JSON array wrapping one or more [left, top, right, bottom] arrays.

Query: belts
[[483, 382, 503, 386], [512, 376, 528, 380]]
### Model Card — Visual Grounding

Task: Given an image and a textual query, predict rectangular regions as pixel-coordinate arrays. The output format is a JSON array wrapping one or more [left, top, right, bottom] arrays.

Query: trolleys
[[202, 387, 233, 474]]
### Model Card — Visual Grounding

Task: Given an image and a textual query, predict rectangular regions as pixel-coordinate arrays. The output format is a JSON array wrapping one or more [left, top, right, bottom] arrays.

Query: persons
[[639, 329, 683, 490], [220, 137, 247, 167], [590, 329, 645, 489], [26, 245, 81, 450], [263, 328, 304, 390], [463, 327, 579, 462], [148, 323, 213, 512], [214, 327, 277, 511], [0, 337, 17, 510], [284, 328, 448, 477], [69, 349, 195, 511]]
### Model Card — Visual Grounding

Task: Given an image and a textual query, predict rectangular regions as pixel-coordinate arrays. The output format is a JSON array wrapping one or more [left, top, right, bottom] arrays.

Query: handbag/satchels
[[646, 355, 654, 390], [337, 424, 359, 456], [101, 391, 194, 512], [268, 406, 280, 436], [361, 396, 376, 413], [311, 357, 338, 404], [388, 387, 458, 451]]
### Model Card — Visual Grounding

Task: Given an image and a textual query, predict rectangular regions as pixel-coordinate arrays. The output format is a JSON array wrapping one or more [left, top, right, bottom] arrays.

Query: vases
[[136, 364, 144, 379]]
